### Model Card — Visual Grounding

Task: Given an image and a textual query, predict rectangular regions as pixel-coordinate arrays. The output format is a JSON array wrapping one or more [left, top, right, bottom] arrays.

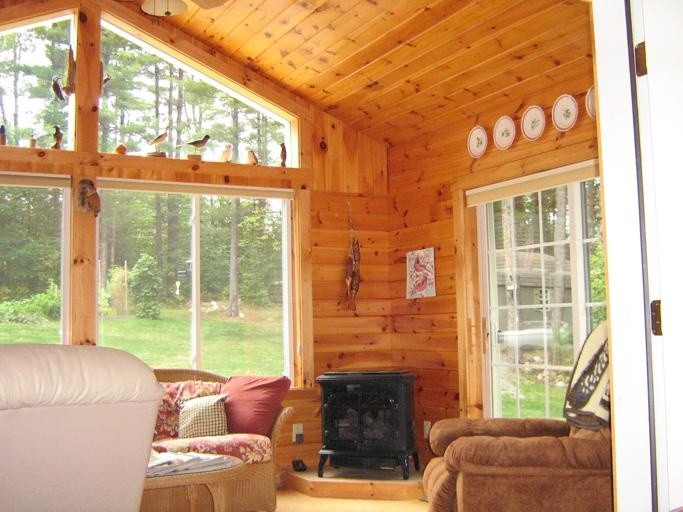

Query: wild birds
[[277, 141, 288, 167], [52, 123, 64, 145], [103, 73, 112, 85], [150, 125, 171, 152], [221, 142, 232, 159], [248, 149, 256, 163], [185, 133, 213, 153], [50, 75, 69, 103], [0, 123, 6, 134]]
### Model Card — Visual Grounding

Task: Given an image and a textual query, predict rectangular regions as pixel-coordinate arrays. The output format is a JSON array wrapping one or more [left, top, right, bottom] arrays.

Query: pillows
[[219, 374, 291, 437], [176, 394, 233, 438], [155, 380, 224, 436]]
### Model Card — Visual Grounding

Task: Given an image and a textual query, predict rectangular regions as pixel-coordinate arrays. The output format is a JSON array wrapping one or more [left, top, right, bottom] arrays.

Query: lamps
[[141, 0, 186, 17]]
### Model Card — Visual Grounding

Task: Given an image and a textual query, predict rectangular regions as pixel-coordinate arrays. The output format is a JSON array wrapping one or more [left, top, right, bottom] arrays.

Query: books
[[146, 448, 232, 479]]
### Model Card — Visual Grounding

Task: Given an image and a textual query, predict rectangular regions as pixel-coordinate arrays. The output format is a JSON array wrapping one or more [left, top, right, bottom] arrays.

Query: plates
[[463, 126, 488, 157], [551, 94, 578, 136], [491, 117, 515, 150], [520, 106, 545, 143], [585, 85, 597, 122]]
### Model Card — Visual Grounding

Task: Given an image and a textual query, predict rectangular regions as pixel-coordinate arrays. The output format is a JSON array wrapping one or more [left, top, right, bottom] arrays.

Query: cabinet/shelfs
[[313, 366, 420, 480]]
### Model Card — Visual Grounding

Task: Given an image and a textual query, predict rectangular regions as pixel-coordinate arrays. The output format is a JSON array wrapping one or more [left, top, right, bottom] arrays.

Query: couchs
[[0, 343, 163, 512], [140, 367, 296, 511], [418, 318, 614, 512]]
